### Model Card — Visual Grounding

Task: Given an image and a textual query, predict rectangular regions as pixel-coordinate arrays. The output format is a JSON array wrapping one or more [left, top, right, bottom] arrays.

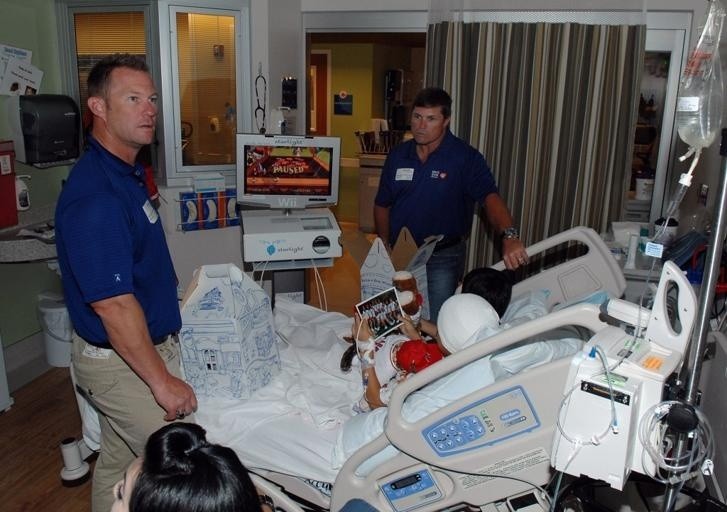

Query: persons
[[372, 333, 443, 387], [25, 87, 34, 94], [348, 292, 501, 411], [8, 82, 22, 92], [372, 85, 533, 341], [108, 421, 277, 510], [53, 50, 199, 509], [416, 265, 514, 343], [355, 296, 401, 336]]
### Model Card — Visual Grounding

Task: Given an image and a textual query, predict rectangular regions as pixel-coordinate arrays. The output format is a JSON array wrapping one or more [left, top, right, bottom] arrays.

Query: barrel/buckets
[[635, 177, 654, 203], [654, 224, 680, 248]]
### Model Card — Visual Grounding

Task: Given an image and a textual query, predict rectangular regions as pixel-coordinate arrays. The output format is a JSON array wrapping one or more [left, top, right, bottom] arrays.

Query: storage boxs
[[177, 262, 282, 403], [360, 228, 445, 321]]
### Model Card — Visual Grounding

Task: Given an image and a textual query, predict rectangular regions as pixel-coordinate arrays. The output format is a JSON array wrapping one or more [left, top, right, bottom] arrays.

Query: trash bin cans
[[37, 289, 72, 369]]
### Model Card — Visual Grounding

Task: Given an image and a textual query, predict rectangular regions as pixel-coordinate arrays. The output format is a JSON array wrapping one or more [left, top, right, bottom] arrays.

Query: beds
[[60, 225, 628, 511]]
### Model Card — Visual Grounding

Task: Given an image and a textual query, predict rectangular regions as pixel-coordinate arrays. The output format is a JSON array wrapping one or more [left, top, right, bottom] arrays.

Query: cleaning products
[[14, 176, 32, 210]]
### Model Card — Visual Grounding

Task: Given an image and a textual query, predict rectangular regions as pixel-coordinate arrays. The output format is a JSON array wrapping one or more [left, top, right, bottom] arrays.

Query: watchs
[[497, 226, 520, 243], [256, 494, 277, 511]]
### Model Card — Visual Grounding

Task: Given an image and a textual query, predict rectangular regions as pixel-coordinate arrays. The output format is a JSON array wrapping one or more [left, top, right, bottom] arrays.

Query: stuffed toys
[[391, 269, 424, 335]]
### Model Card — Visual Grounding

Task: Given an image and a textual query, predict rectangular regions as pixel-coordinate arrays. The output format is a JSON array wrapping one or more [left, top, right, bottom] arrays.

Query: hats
[[437, 293, 500, 353]]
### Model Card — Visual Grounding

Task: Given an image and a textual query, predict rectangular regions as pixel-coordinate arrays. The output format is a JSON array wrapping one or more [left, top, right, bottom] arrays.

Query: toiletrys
[[623, 234, 640, 270]]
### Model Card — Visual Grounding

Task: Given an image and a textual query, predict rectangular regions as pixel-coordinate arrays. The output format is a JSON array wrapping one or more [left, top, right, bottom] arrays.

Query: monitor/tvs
[[235, 132, 341, 209]]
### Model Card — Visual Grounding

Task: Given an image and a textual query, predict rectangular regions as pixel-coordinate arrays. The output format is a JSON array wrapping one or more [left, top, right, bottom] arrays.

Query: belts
[[85, 336, 168, 350]]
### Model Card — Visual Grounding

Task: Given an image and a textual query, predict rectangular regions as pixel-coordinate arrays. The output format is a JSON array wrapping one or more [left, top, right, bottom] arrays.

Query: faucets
[[17, 173, 32, 181]]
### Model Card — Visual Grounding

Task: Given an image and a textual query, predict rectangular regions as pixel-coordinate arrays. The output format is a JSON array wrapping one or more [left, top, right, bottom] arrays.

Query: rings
[[516, 256, 524, 262], [175, 409, 186, 420]]
